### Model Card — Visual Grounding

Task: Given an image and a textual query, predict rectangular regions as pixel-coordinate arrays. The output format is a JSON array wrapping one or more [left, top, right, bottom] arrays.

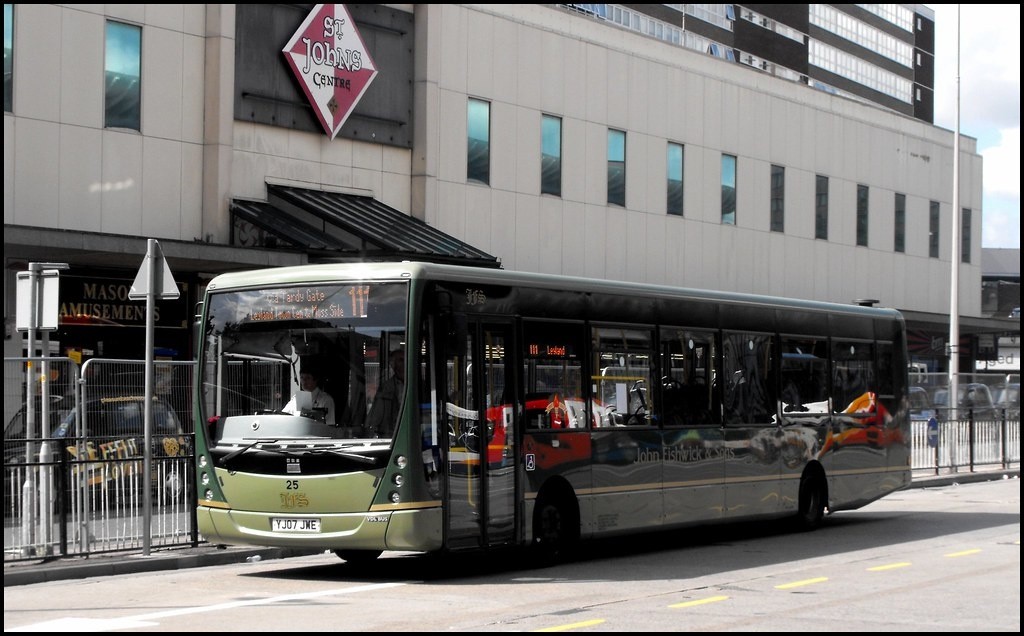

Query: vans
[[3, 391, 191, 516]]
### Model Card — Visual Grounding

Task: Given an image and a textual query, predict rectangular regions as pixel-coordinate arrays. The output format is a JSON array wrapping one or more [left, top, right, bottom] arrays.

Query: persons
[[282, 366, 336, 425], [364, 350, 404, 439]]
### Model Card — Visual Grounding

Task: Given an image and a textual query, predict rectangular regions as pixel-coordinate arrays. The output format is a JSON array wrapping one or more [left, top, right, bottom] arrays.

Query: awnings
[[229, 176, 503, 269]]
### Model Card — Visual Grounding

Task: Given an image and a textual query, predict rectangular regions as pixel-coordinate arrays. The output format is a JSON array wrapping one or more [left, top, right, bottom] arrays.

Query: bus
[[191, 259, 910, 570]]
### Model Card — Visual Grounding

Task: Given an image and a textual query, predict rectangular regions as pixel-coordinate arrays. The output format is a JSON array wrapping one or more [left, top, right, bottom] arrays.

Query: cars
[[911, 382, 1021, 421]]
